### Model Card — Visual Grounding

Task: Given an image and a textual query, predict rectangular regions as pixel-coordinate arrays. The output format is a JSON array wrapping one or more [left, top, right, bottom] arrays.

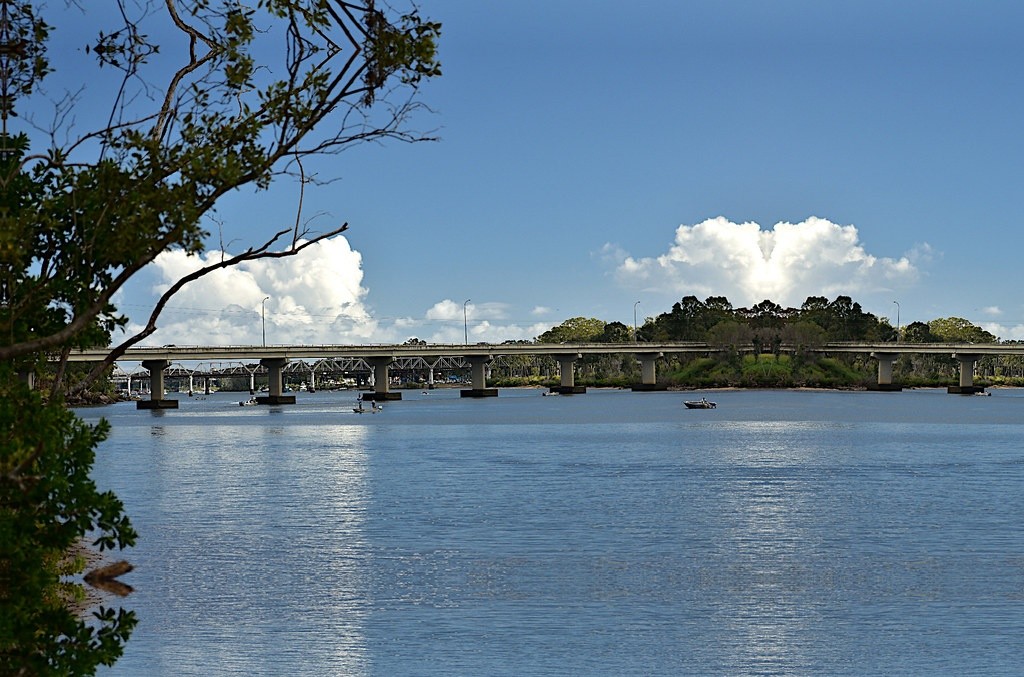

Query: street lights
[[261, 295, 270, 346], [463, 299, 470, 345], [894, 299, 900, 344], [634, 301, 640, 343]]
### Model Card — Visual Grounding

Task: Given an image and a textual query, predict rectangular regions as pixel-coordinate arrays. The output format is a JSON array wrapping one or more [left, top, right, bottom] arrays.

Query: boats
[[974, 390, 993, 397], [680, 397, 718, 410], [542, 390, 561, 397], [238, 395, 258, 407], [353, 392, 382, 415]]
